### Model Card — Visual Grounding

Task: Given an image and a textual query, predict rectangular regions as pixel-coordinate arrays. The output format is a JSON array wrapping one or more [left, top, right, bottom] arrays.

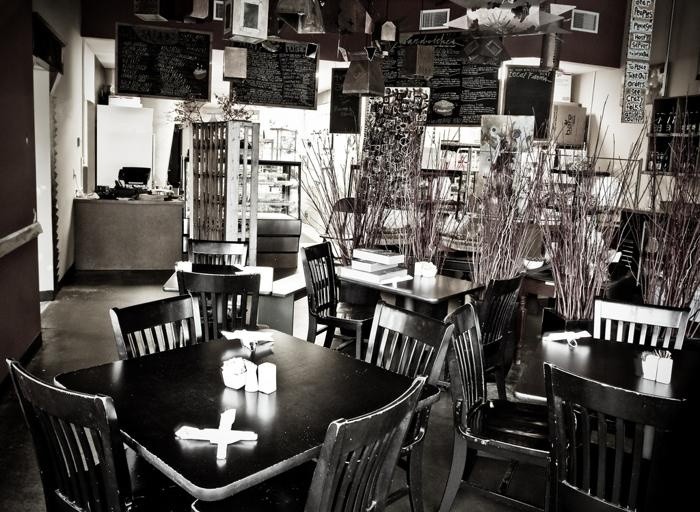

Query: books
[[353, 247, 405, 266], [339, 266, 408, 285], [352, 260, 398, 273]]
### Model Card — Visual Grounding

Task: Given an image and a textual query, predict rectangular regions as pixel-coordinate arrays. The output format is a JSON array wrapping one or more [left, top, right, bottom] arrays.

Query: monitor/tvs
[[119, 166, 151, 185]]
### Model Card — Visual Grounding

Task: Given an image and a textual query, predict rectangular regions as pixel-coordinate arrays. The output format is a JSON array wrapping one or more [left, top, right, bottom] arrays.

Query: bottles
[[647, 109, 700, 171]]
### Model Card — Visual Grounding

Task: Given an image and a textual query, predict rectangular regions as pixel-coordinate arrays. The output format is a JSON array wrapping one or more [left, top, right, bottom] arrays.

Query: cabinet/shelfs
[[641, 94, 700, 177], [182, 155, 303, 282]]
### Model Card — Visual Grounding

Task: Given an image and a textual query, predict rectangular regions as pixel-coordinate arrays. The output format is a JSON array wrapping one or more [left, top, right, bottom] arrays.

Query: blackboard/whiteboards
[[115, 22, 213, 102], [369, 31, 501, 127], [502, 64, 556, 141], [329, 67, 361, 133], [644, 95, 698, 173], [228, 36, 320, 110]]
